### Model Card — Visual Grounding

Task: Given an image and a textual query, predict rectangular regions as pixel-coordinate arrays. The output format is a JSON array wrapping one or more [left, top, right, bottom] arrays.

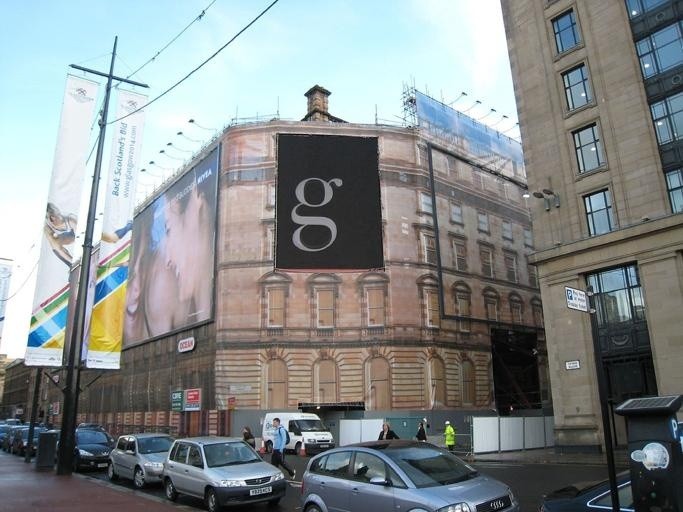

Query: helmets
[[445, 421, 450, 425]]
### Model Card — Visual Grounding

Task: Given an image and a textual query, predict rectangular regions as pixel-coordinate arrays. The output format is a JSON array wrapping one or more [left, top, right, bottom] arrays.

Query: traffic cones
[[298, 438, 308, 458], [259, 439, 267, 456]]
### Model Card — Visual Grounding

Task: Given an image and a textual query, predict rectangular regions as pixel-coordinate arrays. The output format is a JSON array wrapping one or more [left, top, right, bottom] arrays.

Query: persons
[[240, 426, 255, 449], [443, 420, 456, 454], [257, 446, 267, 459], [377, 422, 400, 440], [123, 206, 177, 344], [161, 148, 216, 331], [44, 202, 119, 267], [415, 422, 427, 442], [270, 417, 296, 480]]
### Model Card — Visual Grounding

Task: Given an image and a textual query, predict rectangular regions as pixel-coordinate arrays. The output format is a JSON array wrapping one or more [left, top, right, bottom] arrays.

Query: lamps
[[532, 188, 559, 211]]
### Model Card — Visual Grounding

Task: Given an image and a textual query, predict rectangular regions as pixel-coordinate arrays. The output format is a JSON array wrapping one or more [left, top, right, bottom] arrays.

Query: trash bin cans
[[35, 432, 59, 472]]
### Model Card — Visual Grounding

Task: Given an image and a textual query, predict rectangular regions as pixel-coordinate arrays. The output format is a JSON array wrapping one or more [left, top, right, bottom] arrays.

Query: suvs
[[162, 436, 290, 512], [297, 437, 521, 512]]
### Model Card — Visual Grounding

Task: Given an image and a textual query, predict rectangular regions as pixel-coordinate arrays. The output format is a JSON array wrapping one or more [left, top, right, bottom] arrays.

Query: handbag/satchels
[[279, 427, 290, 445]]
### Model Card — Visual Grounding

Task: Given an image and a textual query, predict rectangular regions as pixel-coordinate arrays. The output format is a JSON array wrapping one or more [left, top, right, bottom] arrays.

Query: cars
[[536, 466, 634, 512], [0, 418, 116, 475], [105, 431, 179, 489]]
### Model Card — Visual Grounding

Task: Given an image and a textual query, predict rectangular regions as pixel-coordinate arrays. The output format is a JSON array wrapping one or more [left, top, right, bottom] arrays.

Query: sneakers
[[290, 469, 296, 480]]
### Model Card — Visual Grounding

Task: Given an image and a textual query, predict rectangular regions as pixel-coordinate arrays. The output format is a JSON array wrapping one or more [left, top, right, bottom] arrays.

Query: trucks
[[261, 410, 337, 455]]
